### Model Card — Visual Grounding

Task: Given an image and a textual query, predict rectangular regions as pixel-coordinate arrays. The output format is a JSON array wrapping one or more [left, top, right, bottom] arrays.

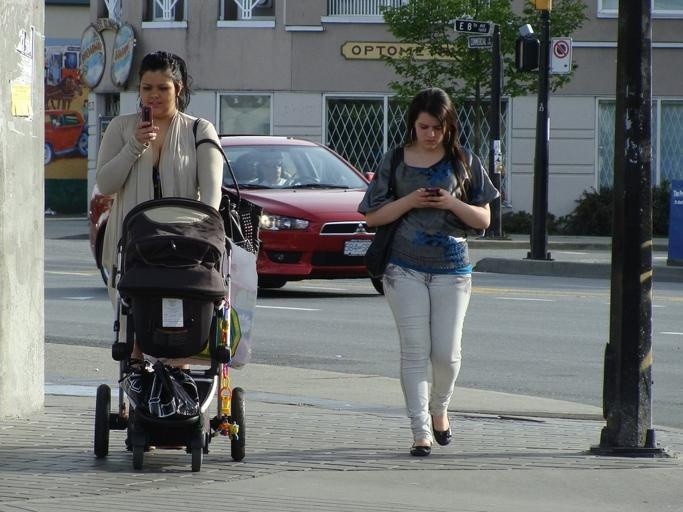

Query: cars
[[90, 131, 401, 303], [44, 107, 87, 165]]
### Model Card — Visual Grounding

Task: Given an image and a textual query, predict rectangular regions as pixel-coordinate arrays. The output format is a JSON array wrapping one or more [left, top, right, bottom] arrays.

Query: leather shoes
[[432, 413, 452, 446], [410, 442, 430, 456]]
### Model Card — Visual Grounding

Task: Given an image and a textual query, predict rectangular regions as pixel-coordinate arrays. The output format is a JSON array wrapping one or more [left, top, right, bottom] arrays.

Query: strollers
[[92, 195, 247, 474]]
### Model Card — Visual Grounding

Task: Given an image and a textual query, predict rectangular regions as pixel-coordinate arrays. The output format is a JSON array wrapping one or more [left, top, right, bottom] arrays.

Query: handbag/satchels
[[363, 148, 400, 278]]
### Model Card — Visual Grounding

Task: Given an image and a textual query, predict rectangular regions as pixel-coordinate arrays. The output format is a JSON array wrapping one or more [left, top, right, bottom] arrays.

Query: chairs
[[257, 163, 284, 184]]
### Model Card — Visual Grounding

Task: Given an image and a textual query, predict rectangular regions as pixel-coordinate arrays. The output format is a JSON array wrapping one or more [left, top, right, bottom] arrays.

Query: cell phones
[[425, 187, 440, 197], [142, 106, 152, 127]]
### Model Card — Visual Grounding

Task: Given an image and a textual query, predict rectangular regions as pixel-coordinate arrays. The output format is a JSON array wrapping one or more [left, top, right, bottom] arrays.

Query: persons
[[96, 49, 223, 451], [356, 88, 503, 459], [258, 149, 298, 188], [234, 152, 259, 181]]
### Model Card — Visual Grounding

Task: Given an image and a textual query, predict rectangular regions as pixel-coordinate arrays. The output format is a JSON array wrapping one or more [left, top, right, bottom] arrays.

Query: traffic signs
[[454, 19, 492, 34], [468, 37, 492, 48]]
